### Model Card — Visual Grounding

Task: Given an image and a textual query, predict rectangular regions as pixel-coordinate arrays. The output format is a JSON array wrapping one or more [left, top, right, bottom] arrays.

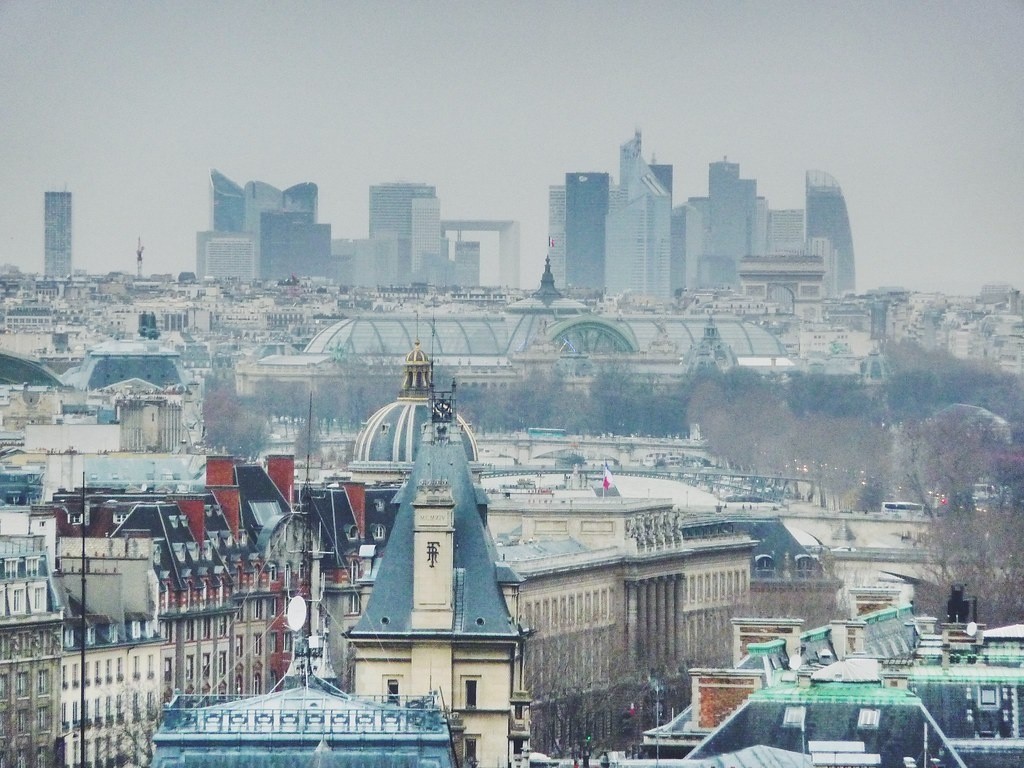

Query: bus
[[882, 502, 926, 519]]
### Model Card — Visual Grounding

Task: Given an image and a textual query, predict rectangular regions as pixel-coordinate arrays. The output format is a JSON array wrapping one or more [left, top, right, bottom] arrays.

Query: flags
[[603, 469, 613, 490], [548, 237, 554, 248], [630, 702, 635, 715]]
